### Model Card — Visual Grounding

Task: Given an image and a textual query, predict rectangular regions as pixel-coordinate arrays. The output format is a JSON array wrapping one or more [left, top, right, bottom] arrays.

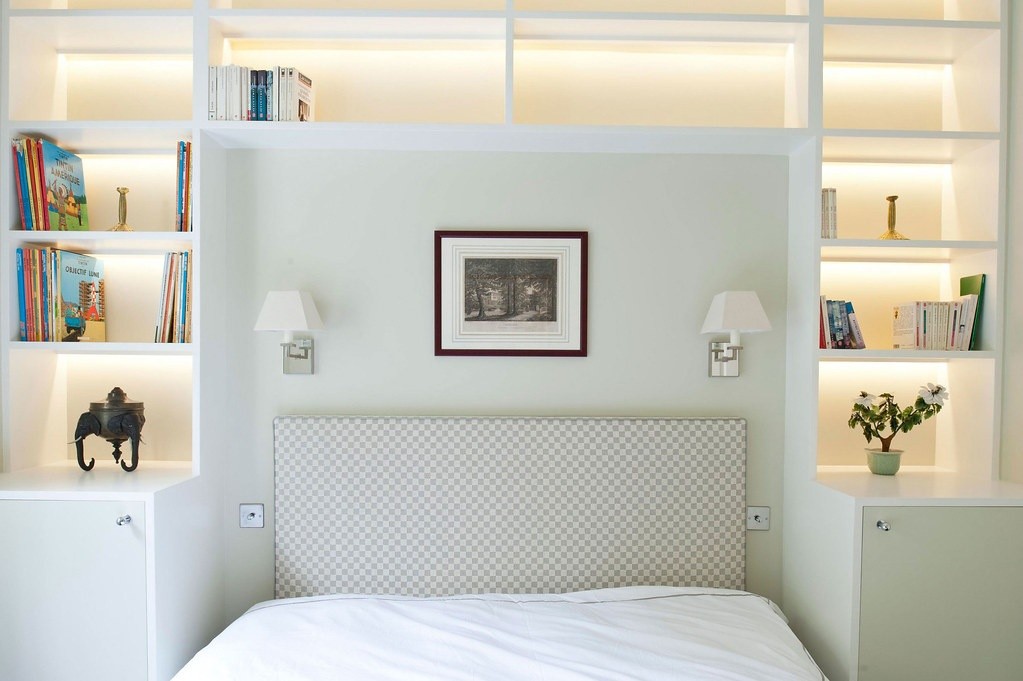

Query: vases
[[864, 448, 904, 475]]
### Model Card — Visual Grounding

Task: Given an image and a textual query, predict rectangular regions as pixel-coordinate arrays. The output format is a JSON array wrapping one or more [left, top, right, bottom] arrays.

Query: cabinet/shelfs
[[0, 0, 1023, 156], [780, 135, 1023, 681], [0, 127, 228, 681]]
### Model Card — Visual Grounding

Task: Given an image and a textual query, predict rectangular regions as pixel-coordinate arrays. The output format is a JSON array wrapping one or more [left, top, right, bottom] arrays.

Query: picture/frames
[[434, 230, 588, 357]]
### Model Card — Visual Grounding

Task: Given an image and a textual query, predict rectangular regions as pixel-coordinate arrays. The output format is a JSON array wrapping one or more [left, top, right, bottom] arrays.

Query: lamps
[[700, 289, 771, 378], [252, 289, 325, 375]]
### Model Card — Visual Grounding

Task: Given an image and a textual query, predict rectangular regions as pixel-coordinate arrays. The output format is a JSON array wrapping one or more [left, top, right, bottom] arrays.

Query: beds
[[171, 416, 831, 681]]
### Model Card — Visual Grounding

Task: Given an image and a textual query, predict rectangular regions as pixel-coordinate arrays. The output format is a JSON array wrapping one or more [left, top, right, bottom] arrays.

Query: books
[[175, 141, 192, 232], [208, 64, 312, 122], [822, 188, 837, 239], [893, 274, 986, 351], [15, 247, 106, 342], [819, 294, 866, 349], [11, 137, 91, 231], [155, 249, 193, 344]]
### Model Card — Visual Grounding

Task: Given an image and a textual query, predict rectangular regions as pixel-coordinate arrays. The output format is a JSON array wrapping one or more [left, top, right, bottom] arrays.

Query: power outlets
[[746, 506, 770, 531], [239, 502, 264, 528]]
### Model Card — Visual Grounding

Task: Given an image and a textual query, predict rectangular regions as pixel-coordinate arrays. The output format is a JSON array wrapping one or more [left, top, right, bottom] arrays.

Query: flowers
[[848, 383, 949, 452]]
